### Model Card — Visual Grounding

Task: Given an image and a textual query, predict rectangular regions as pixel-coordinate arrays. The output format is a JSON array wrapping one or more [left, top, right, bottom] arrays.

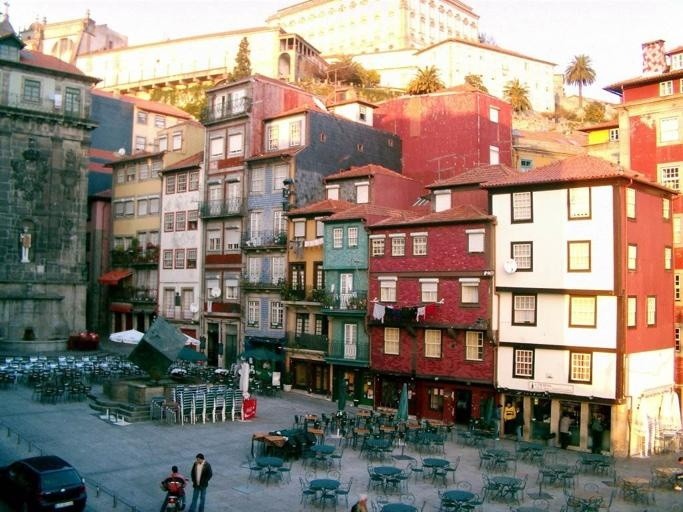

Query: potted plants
[[282, 371, 295, 392]]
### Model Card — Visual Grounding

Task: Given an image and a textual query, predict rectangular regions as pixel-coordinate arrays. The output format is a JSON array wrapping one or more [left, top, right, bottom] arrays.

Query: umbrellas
[[393, 383, 409, 433]]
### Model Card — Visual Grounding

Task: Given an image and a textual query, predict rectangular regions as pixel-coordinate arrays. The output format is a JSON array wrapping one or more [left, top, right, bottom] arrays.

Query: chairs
[[244, 407, 682, 512], [0, 353, 282, 428]]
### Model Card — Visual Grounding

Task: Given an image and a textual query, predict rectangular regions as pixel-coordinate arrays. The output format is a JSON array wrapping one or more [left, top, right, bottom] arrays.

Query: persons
[[589, 415, 608, 454], [559, 413, 575, 450], [351, 494, 368, 512], [188, 453, 212, 512], [672, 457, 683, 490], [513, 408, 525, 443], [160, 466, 188, 512], [503, 402, 516, 433], [494, 403, 503, 440], [19, 226, 31, 263]]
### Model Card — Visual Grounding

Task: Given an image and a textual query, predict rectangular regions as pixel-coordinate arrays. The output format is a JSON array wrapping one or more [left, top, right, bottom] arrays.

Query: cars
[[0, 455, 86, 512]]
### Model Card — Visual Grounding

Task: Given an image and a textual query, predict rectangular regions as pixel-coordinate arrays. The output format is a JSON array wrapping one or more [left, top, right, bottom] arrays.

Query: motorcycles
[[166, 479, 187, 511]]
